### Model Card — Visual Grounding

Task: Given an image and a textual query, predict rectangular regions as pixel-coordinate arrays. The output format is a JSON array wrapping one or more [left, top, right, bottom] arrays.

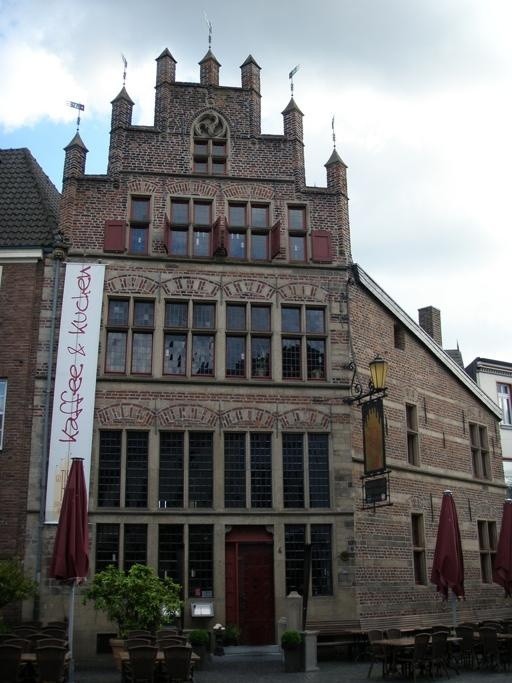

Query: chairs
[[366, 619, 512, 683], [0, 620, 68, 683], [124, 628, 193, 683], [117, 651, 201, 682]]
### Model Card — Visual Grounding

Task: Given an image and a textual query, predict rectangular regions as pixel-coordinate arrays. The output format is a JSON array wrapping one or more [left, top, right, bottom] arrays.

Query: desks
[[344, 628, 383, 661]]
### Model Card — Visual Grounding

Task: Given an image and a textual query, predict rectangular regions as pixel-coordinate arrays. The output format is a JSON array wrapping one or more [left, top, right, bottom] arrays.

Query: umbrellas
[[494, 499, 512, 599], [47, 457, 90, 683], [430, 490, 465, 636]]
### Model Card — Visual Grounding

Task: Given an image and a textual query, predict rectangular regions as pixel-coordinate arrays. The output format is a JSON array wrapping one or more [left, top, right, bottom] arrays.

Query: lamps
[[343, 354, 388, 400]]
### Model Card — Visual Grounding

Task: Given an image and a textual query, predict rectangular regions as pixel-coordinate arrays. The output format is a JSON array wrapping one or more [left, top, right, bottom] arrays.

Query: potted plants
[[190, 628, 211, 665], [281, 630, 302, 672], [80, 563, 186, 672]]
